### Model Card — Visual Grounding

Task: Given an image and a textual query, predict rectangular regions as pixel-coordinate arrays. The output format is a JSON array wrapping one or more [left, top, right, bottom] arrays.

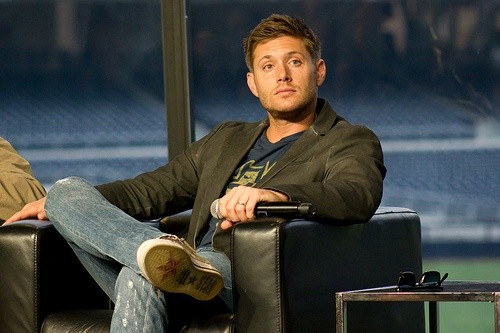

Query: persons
[[0, 136, 48, 221], [1, 13, 388, 333]]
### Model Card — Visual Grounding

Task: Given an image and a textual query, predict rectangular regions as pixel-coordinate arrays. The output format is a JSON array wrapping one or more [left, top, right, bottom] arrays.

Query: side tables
[[335, 282, 500, 333]]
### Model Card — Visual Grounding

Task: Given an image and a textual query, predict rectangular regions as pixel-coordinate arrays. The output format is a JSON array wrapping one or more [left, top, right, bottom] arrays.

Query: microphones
[[210, 199, 313, 219]]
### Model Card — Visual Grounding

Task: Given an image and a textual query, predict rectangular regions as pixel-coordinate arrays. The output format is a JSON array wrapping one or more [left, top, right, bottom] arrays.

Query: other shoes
[[136, 234, 223, 300]]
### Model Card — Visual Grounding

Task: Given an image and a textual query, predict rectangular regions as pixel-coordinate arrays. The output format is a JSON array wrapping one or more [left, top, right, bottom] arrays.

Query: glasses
[[397, 269, 449, 290]]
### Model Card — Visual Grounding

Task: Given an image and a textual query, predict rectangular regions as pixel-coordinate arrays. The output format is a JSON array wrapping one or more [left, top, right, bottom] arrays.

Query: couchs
[[0, 207, 426, 333]]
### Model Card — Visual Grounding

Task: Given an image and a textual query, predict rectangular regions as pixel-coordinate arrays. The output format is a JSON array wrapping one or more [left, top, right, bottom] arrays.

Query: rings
[[238, 202, 244, 206]]
